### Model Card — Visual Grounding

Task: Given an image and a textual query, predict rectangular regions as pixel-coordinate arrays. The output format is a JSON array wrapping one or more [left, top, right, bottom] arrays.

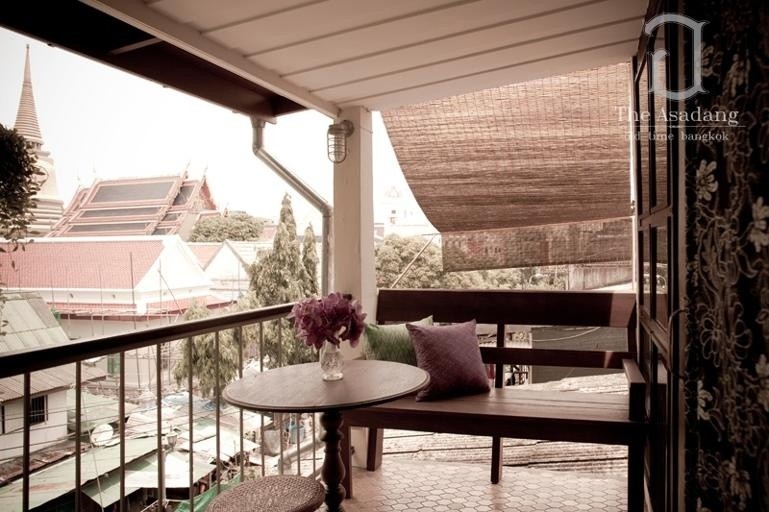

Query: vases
[[319, 330, 344, 381]]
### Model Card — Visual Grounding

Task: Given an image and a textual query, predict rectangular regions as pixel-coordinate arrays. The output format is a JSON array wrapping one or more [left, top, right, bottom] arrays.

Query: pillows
[[365, 317, 491, 402]]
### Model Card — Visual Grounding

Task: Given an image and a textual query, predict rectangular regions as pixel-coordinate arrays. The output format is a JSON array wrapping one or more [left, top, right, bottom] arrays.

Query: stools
[[201, 476, 327, 512]]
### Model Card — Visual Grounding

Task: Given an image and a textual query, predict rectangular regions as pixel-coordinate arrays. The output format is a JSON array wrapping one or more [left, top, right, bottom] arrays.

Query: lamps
[[327, 120, 353, 163]]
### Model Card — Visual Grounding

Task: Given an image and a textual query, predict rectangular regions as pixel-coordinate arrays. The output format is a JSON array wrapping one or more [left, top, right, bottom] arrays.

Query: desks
[[223, 360, 431, 512]]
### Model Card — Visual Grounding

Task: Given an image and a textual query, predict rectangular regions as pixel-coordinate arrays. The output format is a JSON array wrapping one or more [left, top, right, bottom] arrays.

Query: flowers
[[291, 291, 366, 348]]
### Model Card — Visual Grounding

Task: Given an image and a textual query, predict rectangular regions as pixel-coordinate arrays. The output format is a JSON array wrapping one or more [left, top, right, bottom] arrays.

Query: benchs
[[340, 287, 643, 510]]
